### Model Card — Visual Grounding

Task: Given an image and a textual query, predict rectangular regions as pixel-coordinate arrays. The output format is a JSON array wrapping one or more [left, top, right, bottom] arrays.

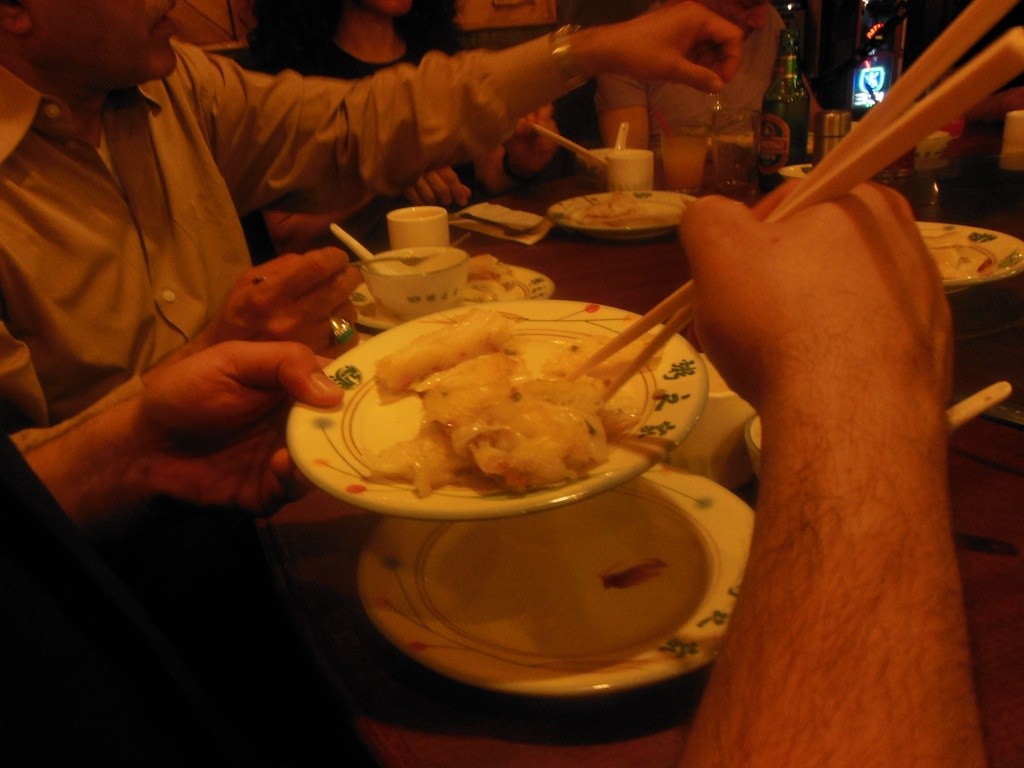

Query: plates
[[548, 191, 697, 241], [778, 163, 814, 184], [347, 263, 556, 332], [913, 220, 1024, 294], [283, 298, 709, 520], [357, 463, 760, 694]]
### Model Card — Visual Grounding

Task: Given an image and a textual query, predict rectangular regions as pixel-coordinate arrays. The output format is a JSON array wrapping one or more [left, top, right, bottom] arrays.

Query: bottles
[[756, 28, 811, 192]]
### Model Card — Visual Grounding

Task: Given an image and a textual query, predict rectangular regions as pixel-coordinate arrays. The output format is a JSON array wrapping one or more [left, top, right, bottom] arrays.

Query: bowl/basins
[[577, 148, 646, 189]]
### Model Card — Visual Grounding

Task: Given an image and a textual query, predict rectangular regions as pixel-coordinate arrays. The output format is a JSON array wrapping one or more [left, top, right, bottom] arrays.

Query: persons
[[595, 0, 823, 148], [2, 0, 745, 426], [261, 0, 472, 256], [1, 173, 990, 767]]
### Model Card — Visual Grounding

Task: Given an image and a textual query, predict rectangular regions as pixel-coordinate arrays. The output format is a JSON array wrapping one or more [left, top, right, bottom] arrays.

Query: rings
[[325, 317, 356, 343]]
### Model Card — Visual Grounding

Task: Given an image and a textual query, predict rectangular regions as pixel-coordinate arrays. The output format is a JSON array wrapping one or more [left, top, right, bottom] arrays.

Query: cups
[[815, 110, 852, 165], [359, 246, 470, 320], [670, 351, 755, 490], [385, 206, 450, 249], [710, 108, 756, 187], [998, 110, 1024, 172], [605, 149, 655, 191], [660, 122, 708, 195]]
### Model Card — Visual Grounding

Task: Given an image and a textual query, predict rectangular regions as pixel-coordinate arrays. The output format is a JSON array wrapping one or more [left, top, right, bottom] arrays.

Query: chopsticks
[[562, 0, 1024, 413], [526, 120, 604, 163]]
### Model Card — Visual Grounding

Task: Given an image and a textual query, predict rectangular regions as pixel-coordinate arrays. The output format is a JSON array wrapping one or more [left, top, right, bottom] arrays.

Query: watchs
[[550, 21, 588, 85]]
